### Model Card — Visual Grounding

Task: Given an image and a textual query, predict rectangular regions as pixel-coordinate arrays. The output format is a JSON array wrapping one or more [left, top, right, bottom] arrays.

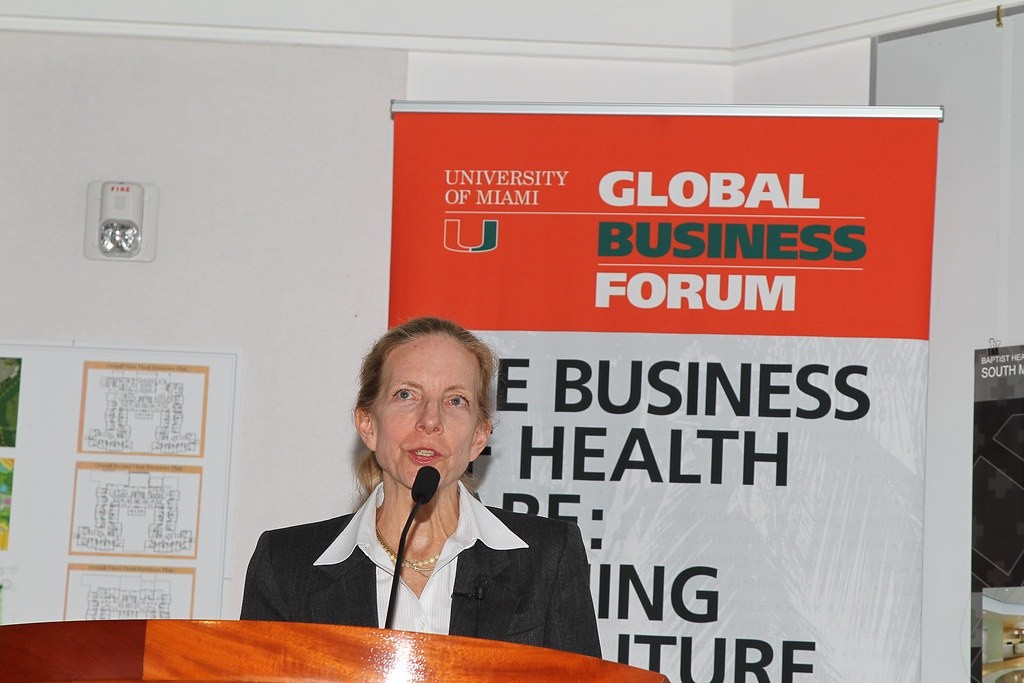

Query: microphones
[[385, 465, 441, 629]]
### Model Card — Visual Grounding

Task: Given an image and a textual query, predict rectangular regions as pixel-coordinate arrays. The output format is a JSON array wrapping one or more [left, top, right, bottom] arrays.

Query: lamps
[[83, 179, 161, 262]]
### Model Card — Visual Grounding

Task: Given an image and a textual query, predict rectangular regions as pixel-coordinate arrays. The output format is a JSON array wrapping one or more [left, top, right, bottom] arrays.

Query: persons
[[238, 316, 602, 661]]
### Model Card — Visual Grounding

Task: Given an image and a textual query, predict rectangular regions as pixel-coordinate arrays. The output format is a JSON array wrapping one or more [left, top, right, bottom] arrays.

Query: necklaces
[[376, 526, 440, 580]]
